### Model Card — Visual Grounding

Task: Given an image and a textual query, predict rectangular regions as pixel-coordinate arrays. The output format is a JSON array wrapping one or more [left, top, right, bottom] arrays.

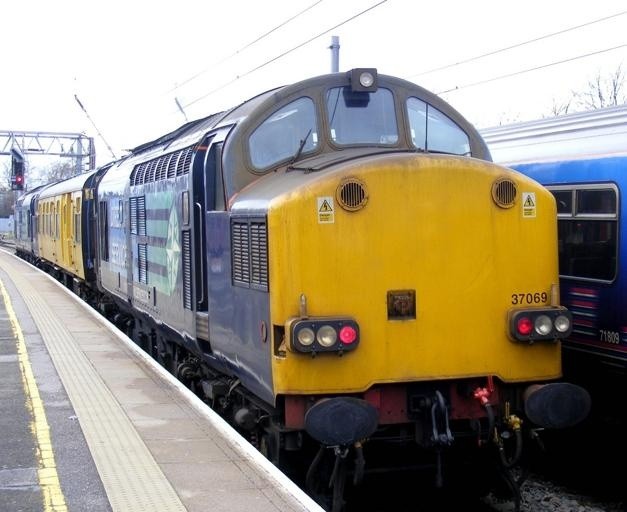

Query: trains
[[465, 103, 626, 371], [11, 67, 591, 511]]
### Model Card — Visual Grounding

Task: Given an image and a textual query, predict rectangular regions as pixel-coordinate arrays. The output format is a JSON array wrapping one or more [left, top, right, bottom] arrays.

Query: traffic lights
[[14, 161, 24, 189]]
